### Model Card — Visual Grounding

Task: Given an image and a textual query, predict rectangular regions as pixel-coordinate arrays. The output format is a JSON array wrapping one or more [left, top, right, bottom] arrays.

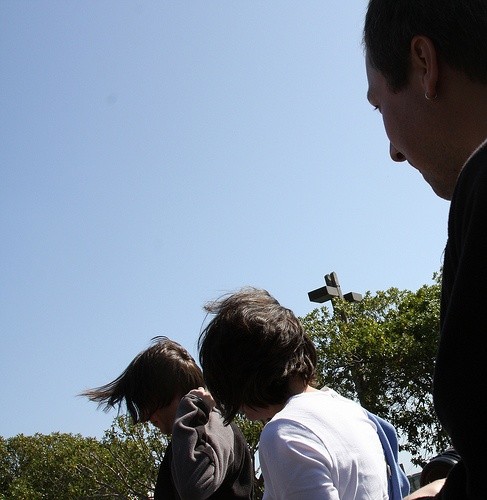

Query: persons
[[363, 1, 486, 500], [75, 336, 253, 500], [195, 286, 410, 500]]
